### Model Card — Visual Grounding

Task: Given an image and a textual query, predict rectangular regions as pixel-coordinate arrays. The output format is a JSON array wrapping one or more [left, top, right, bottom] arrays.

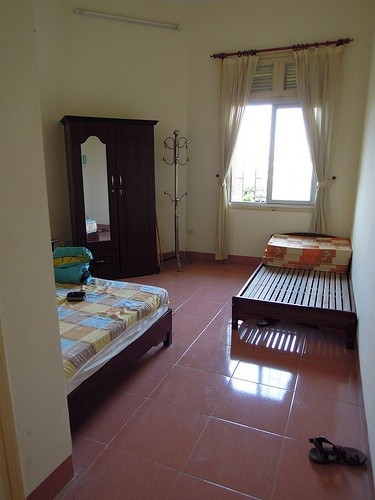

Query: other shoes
[[257, 318, 276, 327]]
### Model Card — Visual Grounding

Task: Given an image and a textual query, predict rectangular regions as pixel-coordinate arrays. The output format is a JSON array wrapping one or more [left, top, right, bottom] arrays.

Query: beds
[[55, 276, 172, 420], [232, 264, 357, 350]]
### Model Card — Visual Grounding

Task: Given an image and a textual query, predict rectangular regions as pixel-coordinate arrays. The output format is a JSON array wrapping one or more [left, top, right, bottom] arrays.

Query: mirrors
[[80, 134, 111, 243]]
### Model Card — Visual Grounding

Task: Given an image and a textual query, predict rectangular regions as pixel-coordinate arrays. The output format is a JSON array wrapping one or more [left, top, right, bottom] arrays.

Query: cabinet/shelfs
[[60, 115, 159, 279]]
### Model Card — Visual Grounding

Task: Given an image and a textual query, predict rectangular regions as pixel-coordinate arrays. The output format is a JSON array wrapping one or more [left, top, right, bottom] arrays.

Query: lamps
[[73, 8, 181, 31]]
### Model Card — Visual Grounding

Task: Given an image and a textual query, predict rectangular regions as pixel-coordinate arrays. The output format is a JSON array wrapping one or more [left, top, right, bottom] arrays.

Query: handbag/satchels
[[53, 247, 94, 285]]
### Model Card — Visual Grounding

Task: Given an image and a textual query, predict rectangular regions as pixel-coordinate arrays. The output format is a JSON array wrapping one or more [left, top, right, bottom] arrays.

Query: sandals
[[308, 436, 366, 466]]
